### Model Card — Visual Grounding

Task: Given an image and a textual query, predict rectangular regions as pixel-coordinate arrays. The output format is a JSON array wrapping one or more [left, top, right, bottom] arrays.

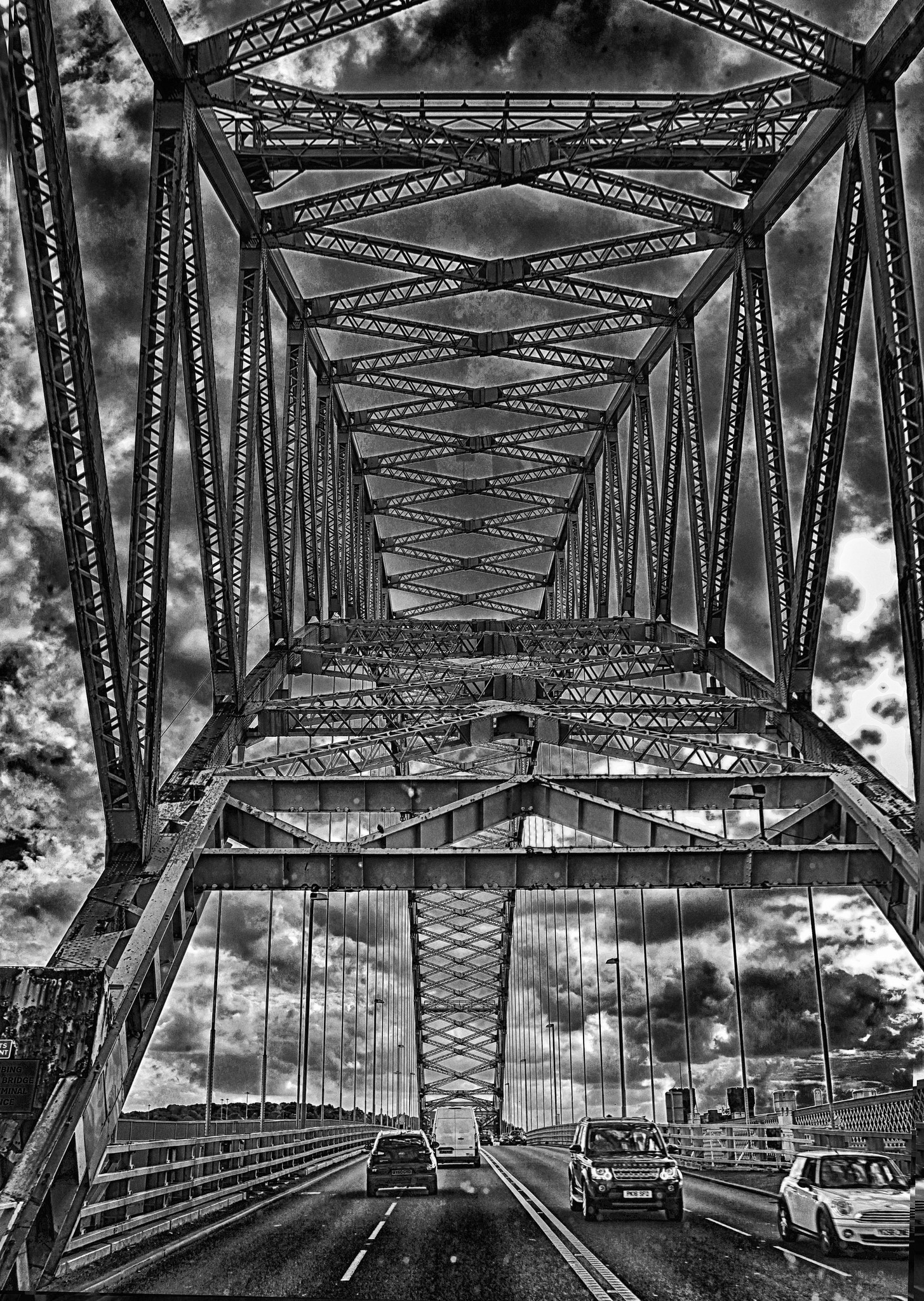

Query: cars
[[508, 1131, 528, 1146], [478, 1130, 495, 1146], [775, 1150, 909, 1261], [424, 1134, 432, 1145], [493, 1138, 501, 1146]]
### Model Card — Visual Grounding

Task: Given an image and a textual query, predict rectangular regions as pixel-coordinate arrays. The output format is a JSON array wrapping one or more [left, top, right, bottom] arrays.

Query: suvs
[[365, 1128, 438, 1195], [567, 1115, 684, 1223], [499, 1133, 511, 1145]]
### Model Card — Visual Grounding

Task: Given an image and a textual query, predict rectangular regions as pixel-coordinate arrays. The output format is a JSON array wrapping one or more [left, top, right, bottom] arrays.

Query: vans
[[430, 1107, 482, 1168]]
